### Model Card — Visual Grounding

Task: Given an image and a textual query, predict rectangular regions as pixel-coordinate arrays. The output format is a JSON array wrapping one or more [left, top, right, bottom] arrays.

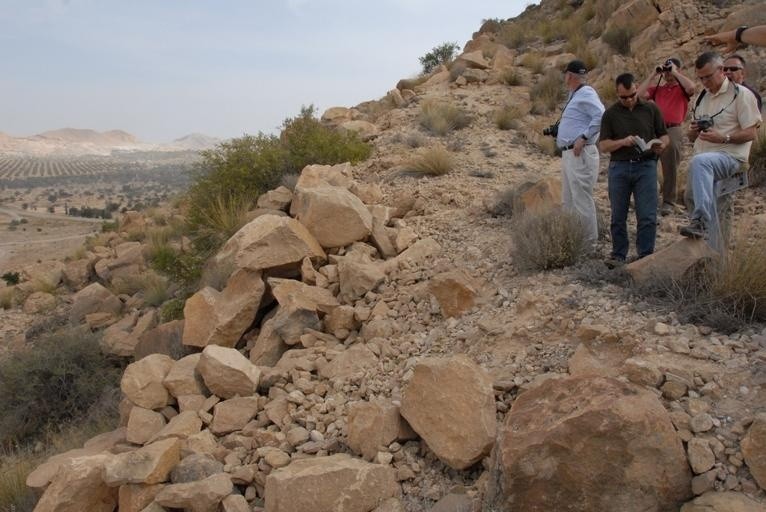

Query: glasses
[[618, 91, 637, 100], [723, 66, 741, 72]]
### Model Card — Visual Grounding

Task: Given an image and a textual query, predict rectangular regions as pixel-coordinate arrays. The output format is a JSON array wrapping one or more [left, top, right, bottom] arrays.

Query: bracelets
[[736, 24, 749, 47], [582, 134, 587, 141], [726, 133, 731, 143]]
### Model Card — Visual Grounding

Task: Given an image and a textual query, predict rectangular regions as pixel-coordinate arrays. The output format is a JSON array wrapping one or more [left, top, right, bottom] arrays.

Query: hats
[[561, 60, 588, 74]]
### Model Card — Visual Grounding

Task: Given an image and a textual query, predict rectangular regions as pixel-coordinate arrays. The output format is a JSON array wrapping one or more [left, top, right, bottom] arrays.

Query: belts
[[666, 122, 680, 128], [559, 145, 574, 150], [610, 157, 641, 162]]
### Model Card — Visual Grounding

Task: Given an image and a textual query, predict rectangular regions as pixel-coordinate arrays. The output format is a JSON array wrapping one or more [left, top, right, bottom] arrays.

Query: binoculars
[[656, 64, 672, 72]]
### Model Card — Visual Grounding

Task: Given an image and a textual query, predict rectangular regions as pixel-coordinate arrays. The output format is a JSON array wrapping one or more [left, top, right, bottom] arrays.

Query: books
[[635, 134, 663, 155]]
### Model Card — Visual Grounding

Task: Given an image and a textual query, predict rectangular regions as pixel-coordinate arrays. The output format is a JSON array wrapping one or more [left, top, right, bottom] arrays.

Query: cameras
[[543, 125, 558, 137], [697, 115, 713, 129], [656, 59, 672, 73]]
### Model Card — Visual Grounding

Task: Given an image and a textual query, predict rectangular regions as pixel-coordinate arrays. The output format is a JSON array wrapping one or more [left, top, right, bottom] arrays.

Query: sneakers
[[604, 259, 625, 266], [680, 220, 704, 237], [662, 203, 674, 216]]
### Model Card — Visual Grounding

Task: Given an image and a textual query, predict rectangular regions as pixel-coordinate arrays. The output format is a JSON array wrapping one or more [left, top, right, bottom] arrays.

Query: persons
[[722, 54, 762, 128], [598, 72, 671, 267], [556, 59, 606, 256], [700, 24, 766, 54], [636, 58, 696, 216], [680, 52, 763, 289]]
